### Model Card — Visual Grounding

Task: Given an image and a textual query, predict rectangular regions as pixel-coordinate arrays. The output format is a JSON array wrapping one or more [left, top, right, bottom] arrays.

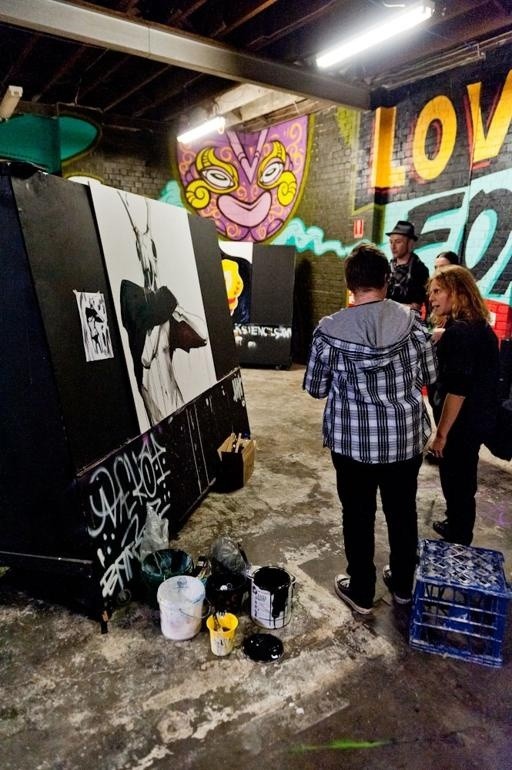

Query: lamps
[[0, 85, 24, 122], [175, 113, 226, 145], [300, 0, 435, 72]]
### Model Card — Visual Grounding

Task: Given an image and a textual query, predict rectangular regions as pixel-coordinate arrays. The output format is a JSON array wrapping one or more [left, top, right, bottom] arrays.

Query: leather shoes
[[433, 520, 447, 535]]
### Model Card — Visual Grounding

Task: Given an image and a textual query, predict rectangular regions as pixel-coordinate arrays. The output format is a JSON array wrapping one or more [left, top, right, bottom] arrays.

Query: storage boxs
[[217, 431, 256, 487]]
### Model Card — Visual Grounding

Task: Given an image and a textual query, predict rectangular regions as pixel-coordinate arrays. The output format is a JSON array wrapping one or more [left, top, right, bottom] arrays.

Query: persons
[[427, 266, 501, 546], [382, 219, 425, 310], [432, 250, 459, 270], [302, 241, 438, 614]]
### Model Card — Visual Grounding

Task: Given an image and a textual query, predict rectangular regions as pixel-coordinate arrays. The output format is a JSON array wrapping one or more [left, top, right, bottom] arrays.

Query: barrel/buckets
[[206, 611, 239, 657], [156, 574, 212, 642], [251, 564, 295, 630], [142, 540, 193, 610], [207, 571, 246, 614]]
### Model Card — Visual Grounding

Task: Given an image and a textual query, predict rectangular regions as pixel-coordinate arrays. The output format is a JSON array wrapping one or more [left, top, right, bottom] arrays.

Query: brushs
[[213, 613, 224, 632]]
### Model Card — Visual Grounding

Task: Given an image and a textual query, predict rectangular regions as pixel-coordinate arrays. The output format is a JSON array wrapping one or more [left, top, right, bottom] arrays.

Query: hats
[[386, 221, 418, 241]]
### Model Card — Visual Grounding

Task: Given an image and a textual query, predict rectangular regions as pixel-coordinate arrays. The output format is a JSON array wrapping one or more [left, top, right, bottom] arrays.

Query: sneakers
[[335, 574, 371, 615], [383, 565, 412, 604]]
